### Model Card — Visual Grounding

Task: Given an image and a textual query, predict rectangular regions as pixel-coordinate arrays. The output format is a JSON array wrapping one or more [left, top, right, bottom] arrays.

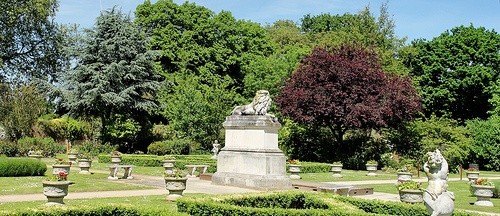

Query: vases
[[28, 155, 43, 161], [52, 164, 71, 176], [288, 164, 301, 179], [42, 180, 71, 206], [466, 171, 495, 206]]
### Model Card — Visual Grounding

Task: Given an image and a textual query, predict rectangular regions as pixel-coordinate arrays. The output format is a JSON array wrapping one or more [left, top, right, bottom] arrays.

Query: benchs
[[185, 164, 210, 176], [107, 164, 136, 179]]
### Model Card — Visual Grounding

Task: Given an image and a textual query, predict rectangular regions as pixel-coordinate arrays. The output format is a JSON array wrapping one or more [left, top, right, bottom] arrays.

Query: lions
[[231, 90, 276, 118], [422, 150, 449, 196]]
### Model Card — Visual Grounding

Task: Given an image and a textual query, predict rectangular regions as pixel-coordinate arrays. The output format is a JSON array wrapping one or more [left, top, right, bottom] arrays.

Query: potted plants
[[160, 154, 188, 198], [395, 169, 423, 205], [110, 150, 123, 164], [365, 160, 378, 175], [68, 149, 92, 174], [330, 161, 343, 177]]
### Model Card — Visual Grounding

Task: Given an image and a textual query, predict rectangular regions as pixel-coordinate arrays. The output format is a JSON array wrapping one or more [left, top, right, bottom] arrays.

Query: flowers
[[467, 167, 476, 171], [473, 178, 495, 186], [288, 159, 301, 165], [44, 171, 67, 182], [52, 159, 72, 165]]
